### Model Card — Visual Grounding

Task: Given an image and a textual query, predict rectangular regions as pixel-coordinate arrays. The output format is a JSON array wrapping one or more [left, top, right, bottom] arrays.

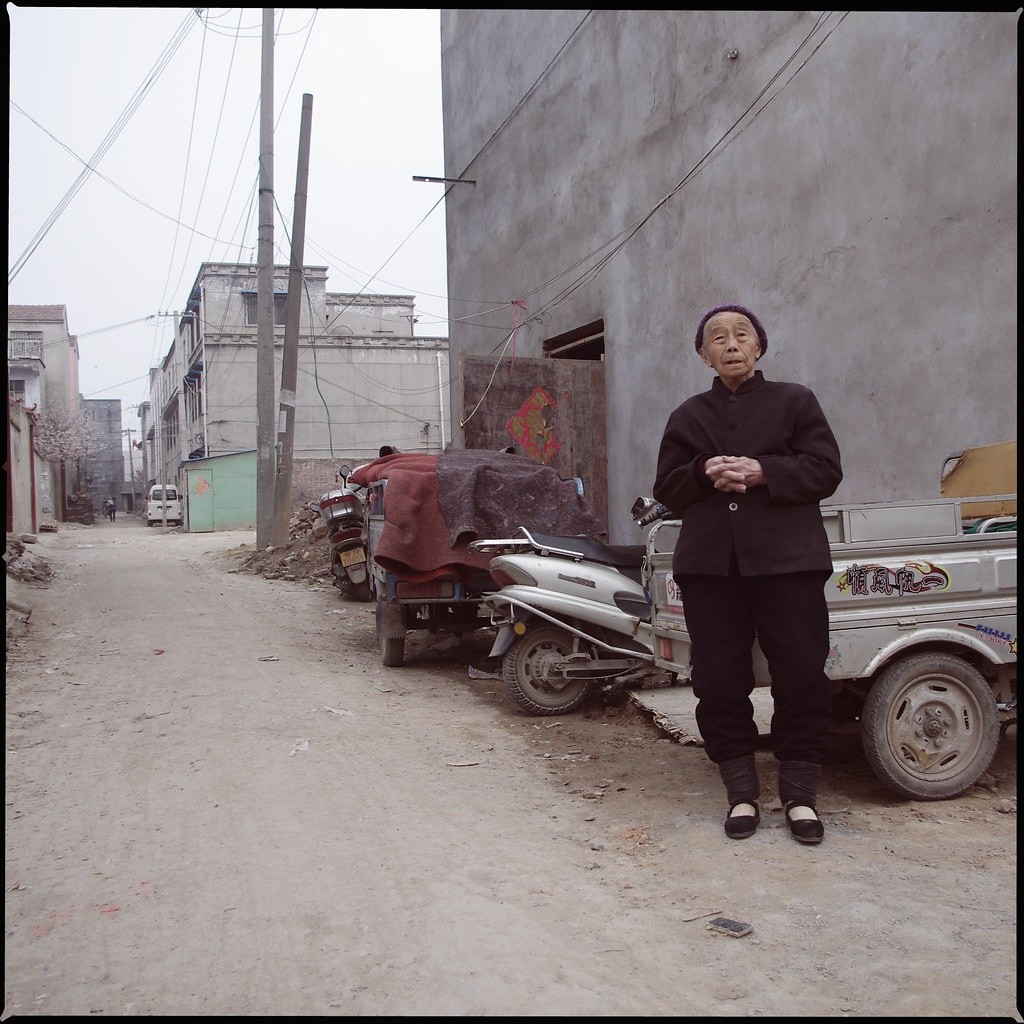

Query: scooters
[[310, 465, 372, 602], [467, 497, 667, 716], [102, 505, 111, 518]]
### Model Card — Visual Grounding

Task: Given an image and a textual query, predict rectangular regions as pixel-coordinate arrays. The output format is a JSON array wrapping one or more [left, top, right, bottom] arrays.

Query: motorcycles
[[641, 452, 1019, 799], [366, 445, 583, 668]]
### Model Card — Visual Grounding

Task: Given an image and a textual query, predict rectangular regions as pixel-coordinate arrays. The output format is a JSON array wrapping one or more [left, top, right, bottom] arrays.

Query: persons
[[655, 304, 844, 846], [108, 497, 117, 522]]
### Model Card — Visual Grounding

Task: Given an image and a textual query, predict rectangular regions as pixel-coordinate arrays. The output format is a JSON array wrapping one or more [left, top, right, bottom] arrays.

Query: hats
[[696, 304, 767, 360]]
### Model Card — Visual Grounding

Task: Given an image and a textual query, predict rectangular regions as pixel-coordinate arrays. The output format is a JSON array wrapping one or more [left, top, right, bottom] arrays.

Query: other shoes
[[786, 802, 825, 842], [724, 800, 760, 838]]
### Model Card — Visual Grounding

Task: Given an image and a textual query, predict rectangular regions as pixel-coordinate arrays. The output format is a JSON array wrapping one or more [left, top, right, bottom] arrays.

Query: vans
[[145, 484, 184, 527]]
